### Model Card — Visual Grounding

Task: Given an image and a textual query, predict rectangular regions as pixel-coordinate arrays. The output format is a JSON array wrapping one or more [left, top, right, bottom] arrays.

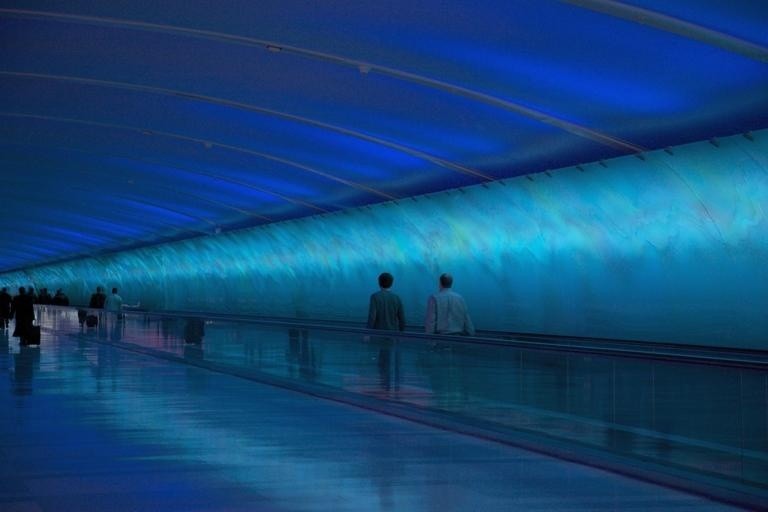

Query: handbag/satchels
[[420, 338, 448, 367]]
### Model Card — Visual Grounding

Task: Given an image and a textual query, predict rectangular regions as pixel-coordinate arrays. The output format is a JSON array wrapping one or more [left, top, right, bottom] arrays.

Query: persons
[[88, 285, 106, 309], [367, 272, 406, 393], [0, 286, 70, 344], [104, 287, 123, 309], [425, 272, 476, 409]]
